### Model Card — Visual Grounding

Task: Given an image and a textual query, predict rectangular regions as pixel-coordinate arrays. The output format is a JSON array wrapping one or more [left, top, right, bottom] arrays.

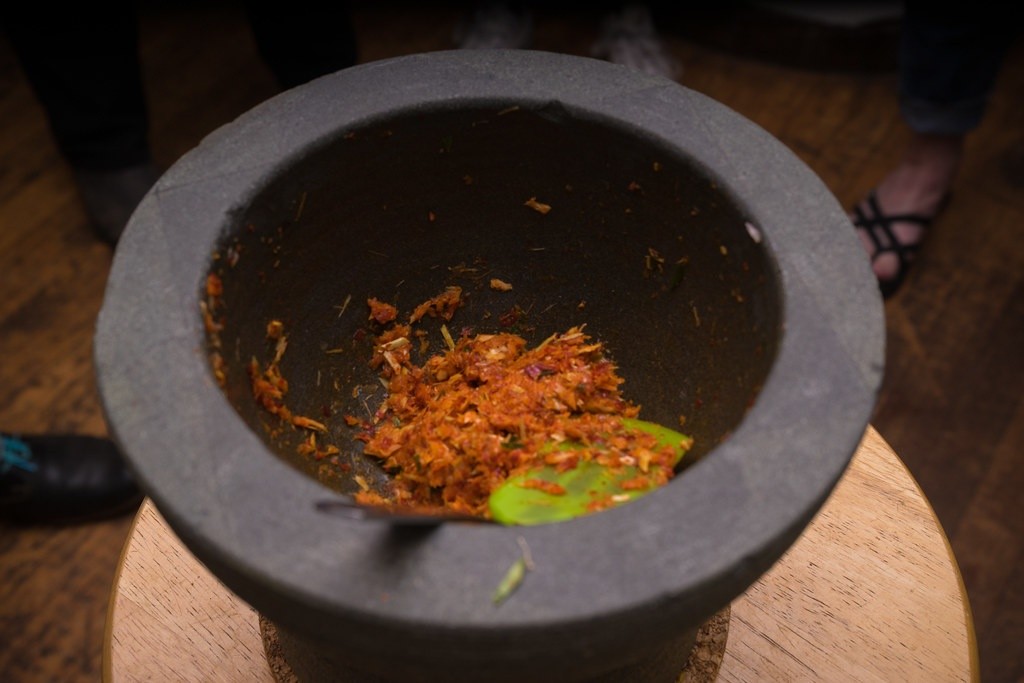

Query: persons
[[847, 0, 1024, 298], [0, 431, 143, 524], [0, 0, 358, 247]]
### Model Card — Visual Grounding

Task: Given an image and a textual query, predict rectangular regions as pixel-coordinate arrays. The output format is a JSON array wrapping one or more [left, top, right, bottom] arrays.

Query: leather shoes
[[0, 432, 143, 531]]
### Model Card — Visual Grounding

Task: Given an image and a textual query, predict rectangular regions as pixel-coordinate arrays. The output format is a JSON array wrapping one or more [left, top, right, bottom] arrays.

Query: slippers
[[845, 174, 957, 300]]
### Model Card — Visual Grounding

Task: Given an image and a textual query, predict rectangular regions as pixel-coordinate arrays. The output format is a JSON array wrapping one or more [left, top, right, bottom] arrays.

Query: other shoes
[[50, 163, 161, 245], [453, 8, 541, 50], [591, 10, 672, 82]]
